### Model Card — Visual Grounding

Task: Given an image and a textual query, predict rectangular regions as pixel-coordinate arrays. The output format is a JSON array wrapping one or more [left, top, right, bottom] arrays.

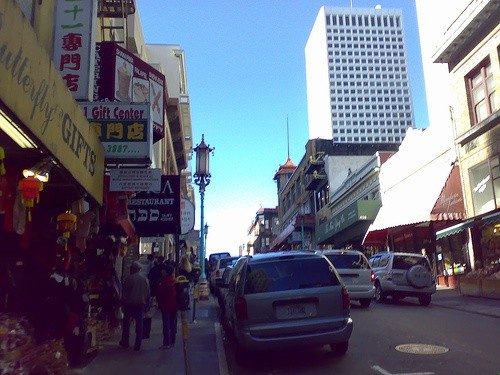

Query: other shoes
[[120, 340, 129, 348], [135, 345, 140, 350], [158, 344, 175, 349]]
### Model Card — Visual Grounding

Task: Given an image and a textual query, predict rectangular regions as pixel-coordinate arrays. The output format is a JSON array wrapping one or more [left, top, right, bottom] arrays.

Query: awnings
[[315, 166, 500, 245]]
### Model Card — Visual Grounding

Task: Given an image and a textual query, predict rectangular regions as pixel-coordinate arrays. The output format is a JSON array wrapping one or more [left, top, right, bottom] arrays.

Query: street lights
[[204, 221, 210, 277], [190, 134, 215, 294], [300, 211, 306, 250]]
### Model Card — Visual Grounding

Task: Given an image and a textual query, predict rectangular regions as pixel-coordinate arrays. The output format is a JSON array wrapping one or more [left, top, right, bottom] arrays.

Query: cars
[[207, 252, 252, 290]]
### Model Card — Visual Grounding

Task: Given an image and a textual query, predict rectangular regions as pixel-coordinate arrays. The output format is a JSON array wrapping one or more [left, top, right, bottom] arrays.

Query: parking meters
[[192, 261, 201, 322], [175, 275, 191, 375]]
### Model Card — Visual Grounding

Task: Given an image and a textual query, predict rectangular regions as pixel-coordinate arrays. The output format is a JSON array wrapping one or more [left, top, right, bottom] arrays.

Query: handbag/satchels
[[143, 313, 152, 339]]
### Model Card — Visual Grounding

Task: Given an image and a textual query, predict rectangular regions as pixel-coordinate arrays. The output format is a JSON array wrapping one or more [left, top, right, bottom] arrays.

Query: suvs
[[368, 252, 436, 307], [222, 249, 354, 356], [316, 249, 377, 309]]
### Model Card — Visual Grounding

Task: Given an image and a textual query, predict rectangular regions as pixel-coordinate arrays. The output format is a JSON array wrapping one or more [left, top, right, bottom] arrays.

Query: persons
[[119, 261, 150, 351], [58, 295, 82, 369], [155, 263, 183, 350], [146, 253, 211, 319]]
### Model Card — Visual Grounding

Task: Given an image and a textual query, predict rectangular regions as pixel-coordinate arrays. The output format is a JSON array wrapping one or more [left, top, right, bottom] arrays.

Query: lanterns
[[17, 176, 43, 222], [56, 209, 78, 251]]
